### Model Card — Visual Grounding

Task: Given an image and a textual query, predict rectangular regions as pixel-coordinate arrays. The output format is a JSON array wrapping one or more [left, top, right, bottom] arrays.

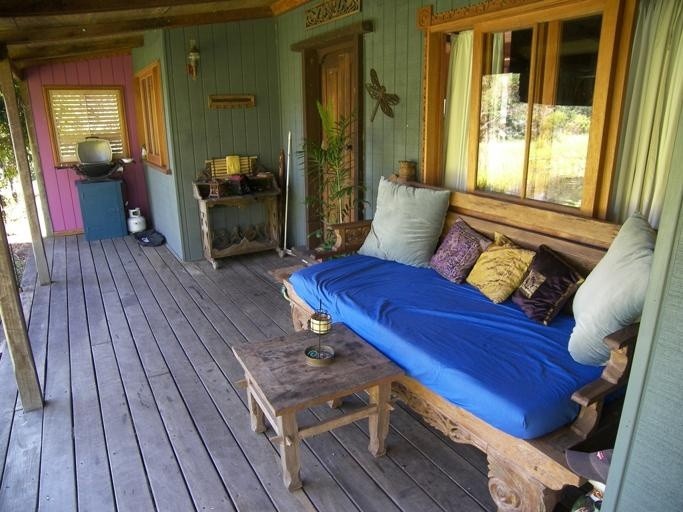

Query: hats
[[565, 448, 614, 484]]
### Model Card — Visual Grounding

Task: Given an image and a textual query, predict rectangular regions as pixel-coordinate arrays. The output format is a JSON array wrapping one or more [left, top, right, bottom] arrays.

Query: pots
[[52, 162, 113, 179]]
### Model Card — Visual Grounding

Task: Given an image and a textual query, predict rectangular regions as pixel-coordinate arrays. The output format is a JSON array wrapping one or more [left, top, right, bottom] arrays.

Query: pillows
[[568, 212, 656, 368], [427, 217, 493, 285], [356, 176, 452, 269], [466, 231, 538, 304], [511, 244, 584, 324]]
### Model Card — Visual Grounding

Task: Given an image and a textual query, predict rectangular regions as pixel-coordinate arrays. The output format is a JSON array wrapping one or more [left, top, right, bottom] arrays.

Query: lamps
[[187, 44, 200, 79]]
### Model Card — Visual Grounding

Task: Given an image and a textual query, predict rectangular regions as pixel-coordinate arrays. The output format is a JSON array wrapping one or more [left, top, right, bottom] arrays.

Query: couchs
[[283, 173, 639, 512]]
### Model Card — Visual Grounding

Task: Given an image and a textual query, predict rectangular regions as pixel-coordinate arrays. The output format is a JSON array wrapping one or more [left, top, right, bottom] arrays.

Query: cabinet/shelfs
[[191, 172, 284, 271], [77, 176, 129, 241]]
